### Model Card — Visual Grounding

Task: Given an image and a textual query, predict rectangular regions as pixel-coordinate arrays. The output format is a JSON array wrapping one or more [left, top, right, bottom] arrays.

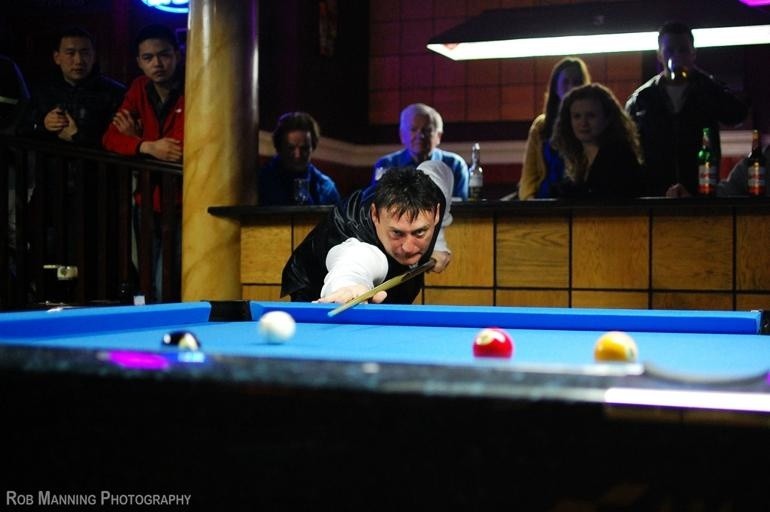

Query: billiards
[[259, 311, 293, 343], [473, 329, 512, 359], [595, 333, 636, 365], [163, 329, 197, 352]]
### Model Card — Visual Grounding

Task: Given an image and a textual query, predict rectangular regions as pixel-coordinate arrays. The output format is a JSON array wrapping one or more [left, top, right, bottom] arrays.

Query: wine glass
[[293, 178, 310, 206]]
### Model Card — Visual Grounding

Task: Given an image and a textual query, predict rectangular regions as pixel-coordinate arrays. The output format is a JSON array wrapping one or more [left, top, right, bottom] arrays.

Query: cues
[[327, 259, 435, 318]]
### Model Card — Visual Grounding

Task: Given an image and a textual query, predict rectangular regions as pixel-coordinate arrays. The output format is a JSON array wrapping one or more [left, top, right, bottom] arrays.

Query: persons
[[665, 106, 770, 199], [624, 20, 721, 198], [254, 110, 341, 205], [103, 25, 185, 304], [25, 30, 139, 307], [549, 85, 666, 204], [371, 104, 470, 199], [519, 58, 591, 199], [280, 159, 455, 304]]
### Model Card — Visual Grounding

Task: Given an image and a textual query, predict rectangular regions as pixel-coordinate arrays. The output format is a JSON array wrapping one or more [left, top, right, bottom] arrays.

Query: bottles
[[468, 142, 484, 205], [696, 127, 718, 198], [745, 128, 769, 197]]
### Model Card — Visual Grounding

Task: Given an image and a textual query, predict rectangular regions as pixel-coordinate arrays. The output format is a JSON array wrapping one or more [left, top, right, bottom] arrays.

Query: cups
[[670, 63, 689, 81]]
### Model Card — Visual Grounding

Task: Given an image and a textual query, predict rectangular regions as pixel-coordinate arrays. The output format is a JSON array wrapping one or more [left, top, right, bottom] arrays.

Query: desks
[[0, 297, 769, 510]]
